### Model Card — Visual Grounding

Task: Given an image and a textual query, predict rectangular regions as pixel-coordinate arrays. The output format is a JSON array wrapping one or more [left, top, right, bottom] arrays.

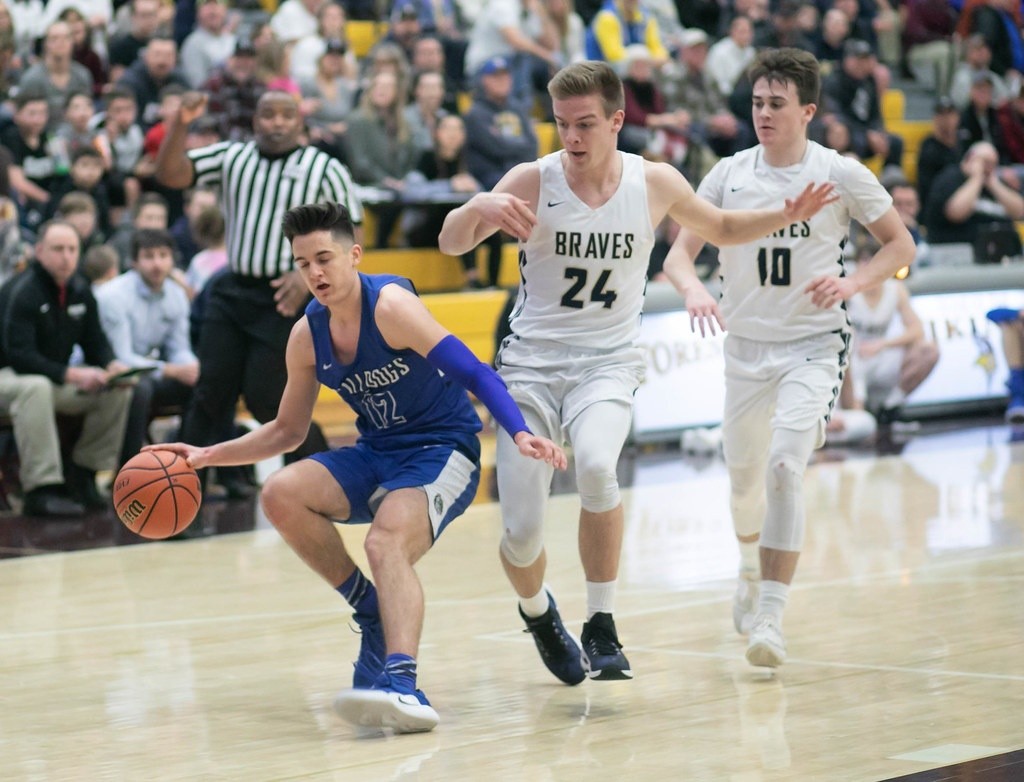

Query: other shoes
[[73, 480, 108, 509], [22, 493, 84, 517], [878, 404, 917, 430], [1006, 396, 1024, 420]]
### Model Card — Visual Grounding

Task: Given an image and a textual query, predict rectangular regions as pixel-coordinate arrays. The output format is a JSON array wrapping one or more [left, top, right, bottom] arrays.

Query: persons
[[826, 242, 940, 456], [0, 0, 1024, 366], [0, 219, 139, 518], [437, 60, 840, 686], [152, 89, 366, 495], [662, 49, 917, 668], [140, 200, 568, 735], [70, 228, 201, 468]]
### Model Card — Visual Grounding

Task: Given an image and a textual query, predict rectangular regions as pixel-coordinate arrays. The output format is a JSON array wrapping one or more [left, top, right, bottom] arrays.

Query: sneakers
[[349, 589, 386, 689], [734, 580, 762, 633], [518, 589, 590, 686], [338, 676, 440, 732], [745, 619, 786, 666], [581, 612, 633, 680]]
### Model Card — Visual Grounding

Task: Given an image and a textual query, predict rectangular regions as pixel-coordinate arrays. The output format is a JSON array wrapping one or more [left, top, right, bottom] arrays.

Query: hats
[[681, 27, 706, 47], [477, 56, 512, 76], [843, 39, 872, 56]]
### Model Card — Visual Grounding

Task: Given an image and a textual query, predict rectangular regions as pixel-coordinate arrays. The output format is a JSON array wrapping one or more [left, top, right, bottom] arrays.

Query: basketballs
[[113, 449, 203, 539]]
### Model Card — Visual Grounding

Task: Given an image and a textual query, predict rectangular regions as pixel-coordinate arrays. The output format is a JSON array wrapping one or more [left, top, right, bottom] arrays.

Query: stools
[[0, 410, 186, 519]]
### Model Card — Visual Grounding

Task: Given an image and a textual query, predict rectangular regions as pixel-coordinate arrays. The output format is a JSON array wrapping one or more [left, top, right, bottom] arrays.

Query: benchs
[[309, 24, 1024, 405]]
